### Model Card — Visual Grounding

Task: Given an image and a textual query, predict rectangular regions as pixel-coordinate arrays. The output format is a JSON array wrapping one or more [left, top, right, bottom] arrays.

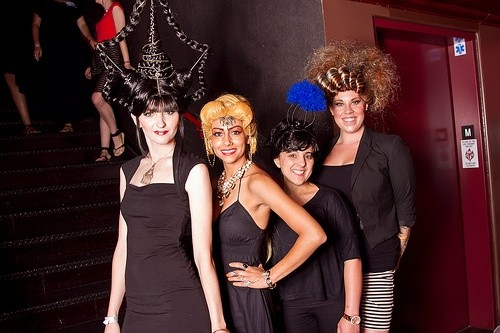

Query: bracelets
[[265, 269, 277, 290], [103, 316, 121, 325]]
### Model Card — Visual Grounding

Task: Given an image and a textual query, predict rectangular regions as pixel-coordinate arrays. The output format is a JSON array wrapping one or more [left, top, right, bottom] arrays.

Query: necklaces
[[140, 143, 175, 185], [216, 158, 252, 205]]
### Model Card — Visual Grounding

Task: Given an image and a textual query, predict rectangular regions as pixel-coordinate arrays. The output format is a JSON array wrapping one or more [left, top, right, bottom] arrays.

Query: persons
[[31, 1, 97, 133], [309, 40, 416, 333], [94, 0, 227, 333], [264, 102, 363, 333], [199, 94, 328, 333], [0, 0, 34, 135], [84, 0, 136, 163]]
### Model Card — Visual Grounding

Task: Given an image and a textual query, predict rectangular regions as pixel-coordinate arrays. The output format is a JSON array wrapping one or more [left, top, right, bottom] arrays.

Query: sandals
[[60, 124, 73, 132], [25, 124, 43, 134], [94, 147, 112, 161], [111, 128, 125, 156]]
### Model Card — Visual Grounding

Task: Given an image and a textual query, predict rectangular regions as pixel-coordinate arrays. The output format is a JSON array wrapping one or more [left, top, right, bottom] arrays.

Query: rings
[[242, 262, 249, 270]]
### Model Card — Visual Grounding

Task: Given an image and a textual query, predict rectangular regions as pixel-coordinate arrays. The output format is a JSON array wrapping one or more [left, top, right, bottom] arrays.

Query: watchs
[[343, 312, 362, 326]]
